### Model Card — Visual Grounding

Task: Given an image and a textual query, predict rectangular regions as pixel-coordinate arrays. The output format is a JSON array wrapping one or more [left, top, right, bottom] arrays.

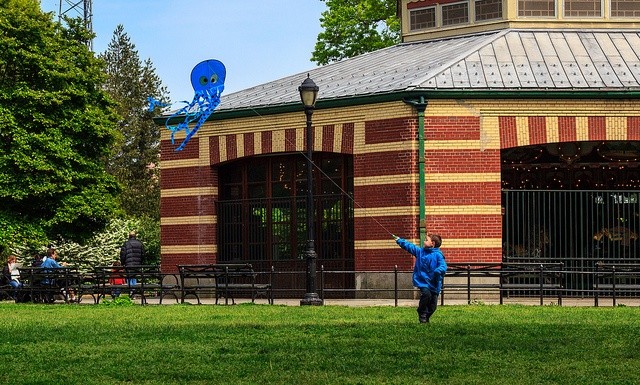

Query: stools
[[441, 261, 566, 310], [588, 262, 640, 306], [178, 262, 273, 307], [15, 267, 82, 301], [93, 265, 176, 306]]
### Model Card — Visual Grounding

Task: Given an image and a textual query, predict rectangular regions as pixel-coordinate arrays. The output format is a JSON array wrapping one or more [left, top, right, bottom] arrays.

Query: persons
[[3, 256, 22, 302], [109, 261, 125, 299], [120, 231, 146, 300], [41, 249, 75, 301], [31, 254, 42, 273], [394, 233, 447, 325]]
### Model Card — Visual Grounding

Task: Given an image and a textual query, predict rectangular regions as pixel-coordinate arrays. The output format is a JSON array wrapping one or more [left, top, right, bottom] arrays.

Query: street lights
[[298, 73, 322, 306]]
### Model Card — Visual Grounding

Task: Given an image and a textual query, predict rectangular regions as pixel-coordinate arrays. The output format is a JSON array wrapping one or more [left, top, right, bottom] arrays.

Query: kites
[[146, 59, 226, 151]]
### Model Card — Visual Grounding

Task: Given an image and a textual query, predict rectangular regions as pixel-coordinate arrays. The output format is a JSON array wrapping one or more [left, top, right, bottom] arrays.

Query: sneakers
[[419, 315, 427, 323]]
